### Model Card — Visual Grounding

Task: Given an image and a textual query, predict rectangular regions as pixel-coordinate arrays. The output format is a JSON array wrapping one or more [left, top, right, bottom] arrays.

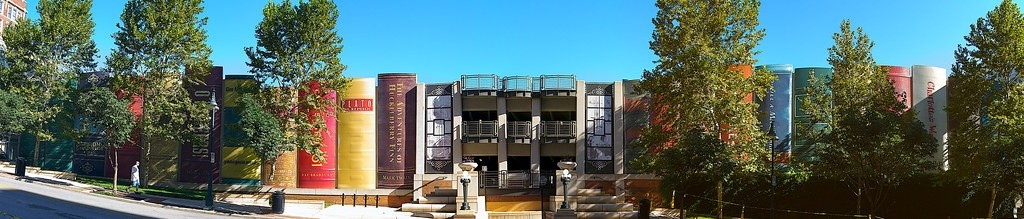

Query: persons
[[125, 161, 140, 194]]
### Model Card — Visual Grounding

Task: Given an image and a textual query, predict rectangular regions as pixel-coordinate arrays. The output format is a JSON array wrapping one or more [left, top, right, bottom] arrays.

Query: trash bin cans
[[15, 157, 27, 176], [638, 199, 650, 219], [272, 191, 286, 214]]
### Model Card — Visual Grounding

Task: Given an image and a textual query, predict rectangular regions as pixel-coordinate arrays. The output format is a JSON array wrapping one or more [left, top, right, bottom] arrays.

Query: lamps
[[460, 171, 471, 180], [562, 169, 571, 179]]
